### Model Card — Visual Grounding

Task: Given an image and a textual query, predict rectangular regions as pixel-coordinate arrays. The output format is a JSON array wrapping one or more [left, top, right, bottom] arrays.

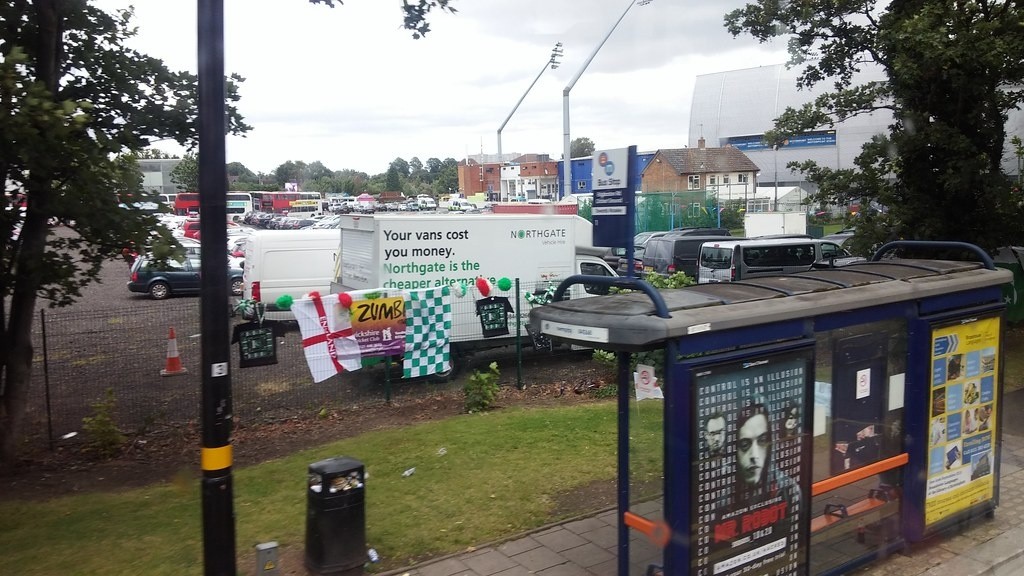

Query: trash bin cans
[[993, 246, 1024, 329], [305, 456, 369, 576]]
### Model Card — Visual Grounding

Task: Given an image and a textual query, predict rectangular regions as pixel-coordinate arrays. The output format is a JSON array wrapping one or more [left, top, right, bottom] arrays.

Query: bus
[[174, 192, 263, 223], [248, 193, 322, 219], [3, 192, 61, 228], [114, 193, 178, 215]]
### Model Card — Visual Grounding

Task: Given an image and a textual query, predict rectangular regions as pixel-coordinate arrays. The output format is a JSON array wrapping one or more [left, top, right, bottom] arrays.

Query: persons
[[784, 406, 798, 438], [704, 416, 727, 459], [978, 406, 992, 431], [719, 393, 802, 508], [964, 409, 975, 433]]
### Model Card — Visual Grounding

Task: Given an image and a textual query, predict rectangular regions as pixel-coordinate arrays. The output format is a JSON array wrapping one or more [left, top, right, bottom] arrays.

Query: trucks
[[241, 231, 341, 321], [329, 214, 632, 385]]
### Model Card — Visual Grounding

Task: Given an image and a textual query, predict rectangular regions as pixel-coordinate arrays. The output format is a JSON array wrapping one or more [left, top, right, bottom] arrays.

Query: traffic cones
[[159, 328, 188, 377]]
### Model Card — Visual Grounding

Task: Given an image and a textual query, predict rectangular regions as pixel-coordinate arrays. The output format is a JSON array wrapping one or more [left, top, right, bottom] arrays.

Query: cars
[[121, 188, 491, 301], [806, 234, 855, 257], [614, 231, 670, 255]]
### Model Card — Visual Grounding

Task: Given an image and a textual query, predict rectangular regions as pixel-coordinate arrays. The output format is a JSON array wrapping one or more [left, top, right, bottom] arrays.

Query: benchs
[[621, 452, 909, 576]]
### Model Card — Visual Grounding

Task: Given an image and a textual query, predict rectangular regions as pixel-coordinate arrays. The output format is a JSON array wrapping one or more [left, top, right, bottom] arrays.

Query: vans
[[640, 237, 755, 282], [510, 198, 526, 202], [528, 199, 551, 205], [698, 239, 866, 284]]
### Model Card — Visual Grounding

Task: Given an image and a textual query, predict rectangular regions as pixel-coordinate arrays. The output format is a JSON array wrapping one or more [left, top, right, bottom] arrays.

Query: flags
[[290, 294, 364, 383], [402, 285, 452, 379]]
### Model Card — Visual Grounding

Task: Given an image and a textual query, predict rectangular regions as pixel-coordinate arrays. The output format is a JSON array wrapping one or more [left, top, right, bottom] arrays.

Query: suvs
[[616, 226, 731, 277]]
